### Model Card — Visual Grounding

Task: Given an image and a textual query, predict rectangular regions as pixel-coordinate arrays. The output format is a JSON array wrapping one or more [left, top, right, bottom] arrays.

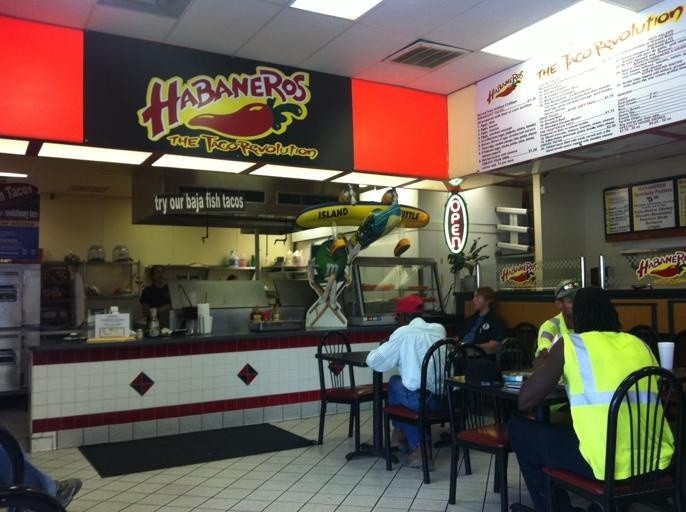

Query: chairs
[[315, 323, 685, 512]]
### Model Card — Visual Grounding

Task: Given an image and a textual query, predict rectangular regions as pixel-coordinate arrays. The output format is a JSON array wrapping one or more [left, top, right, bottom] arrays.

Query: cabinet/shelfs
[[42, 256, 145, 336]]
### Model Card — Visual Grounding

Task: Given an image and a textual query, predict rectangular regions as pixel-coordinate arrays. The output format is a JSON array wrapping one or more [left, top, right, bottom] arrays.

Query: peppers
[[510, 271, 534, 282], [188, 98, 302, 138], [496, 80, 520, 97], [651, 263, 686, 276]]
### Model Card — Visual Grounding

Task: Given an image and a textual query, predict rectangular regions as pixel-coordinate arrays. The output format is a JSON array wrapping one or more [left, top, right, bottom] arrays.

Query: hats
[[396, 295, 424, 316], [552, 276, 584, 299]]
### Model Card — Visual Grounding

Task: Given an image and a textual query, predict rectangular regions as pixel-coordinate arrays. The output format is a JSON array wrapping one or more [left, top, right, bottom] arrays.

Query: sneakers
[[409, 446, 434, 471], [54, 475, 83, 508]]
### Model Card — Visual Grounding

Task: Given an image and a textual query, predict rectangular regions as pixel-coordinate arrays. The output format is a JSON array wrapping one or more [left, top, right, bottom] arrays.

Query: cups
[[657, 341, 675, 370]]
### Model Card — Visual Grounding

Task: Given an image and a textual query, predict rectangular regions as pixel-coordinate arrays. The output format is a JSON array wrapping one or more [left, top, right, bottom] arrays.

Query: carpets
[[78, 423, 314, 478]]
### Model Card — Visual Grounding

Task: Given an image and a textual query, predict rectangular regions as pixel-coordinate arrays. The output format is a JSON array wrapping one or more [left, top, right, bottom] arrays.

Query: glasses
[[556, 279, 579, 294]]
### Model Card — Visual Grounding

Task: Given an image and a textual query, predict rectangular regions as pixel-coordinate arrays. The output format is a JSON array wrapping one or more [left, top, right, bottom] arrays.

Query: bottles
[[113, 244, 129, 261], [145, 307, 161, 338], [88, 245, 106, 261]]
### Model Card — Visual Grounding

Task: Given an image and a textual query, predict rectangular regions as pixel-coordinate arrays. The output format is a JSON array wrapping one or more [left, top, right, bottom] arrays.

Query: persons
[[365, 296, 447, 470], [140, 265, 171, 329], [434, 286, 504, 440], [507, 286, 676, 512], [0, 445, 81, 512], [532, 279, 581, 413]]
